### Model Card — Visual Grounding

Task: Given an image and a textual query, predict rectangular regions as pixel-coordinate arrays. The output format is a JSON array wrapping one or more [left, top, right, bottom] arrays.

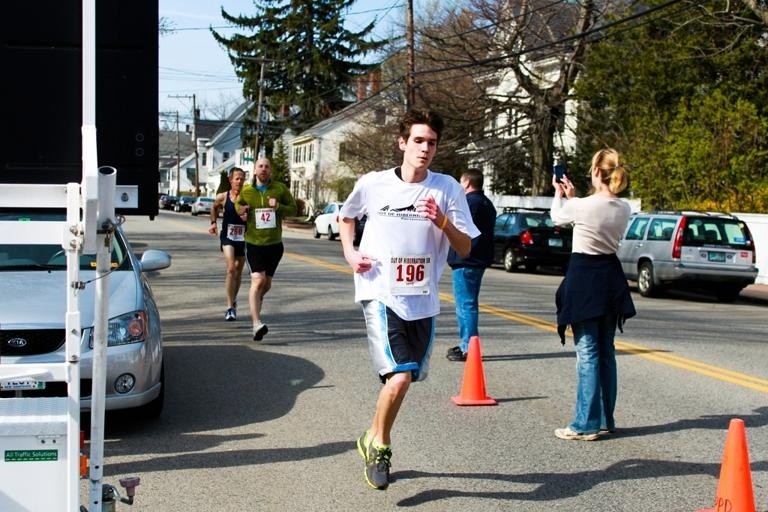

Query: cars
[[311, 202, 354, 240], [0, 198, 176, 412], [157, 190, 221, 216], [483, 202, 572, 275]]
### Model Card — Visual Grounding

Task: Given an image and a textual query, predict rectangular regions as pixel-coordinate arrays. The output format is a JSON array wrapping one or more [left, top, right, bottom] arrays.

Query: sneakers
[[553, 425, 600, 441], [253, 322, 267, 343], [222, 301, 238, 320], [356, 427, 395, 491], [598, 424, 616, 436]]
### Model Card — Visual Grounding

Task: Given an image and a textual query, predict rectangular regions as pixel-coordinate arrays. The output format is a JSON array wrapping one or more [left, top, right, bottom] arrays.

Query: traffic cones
[[450, 333, 500, 414], [696, 414, 762, 510]]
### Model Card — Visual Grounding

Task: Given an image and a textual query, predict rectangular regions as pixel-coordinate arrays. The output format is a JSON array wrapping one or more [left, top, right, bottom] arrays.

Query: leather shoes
[[444, 347, 470, 362]]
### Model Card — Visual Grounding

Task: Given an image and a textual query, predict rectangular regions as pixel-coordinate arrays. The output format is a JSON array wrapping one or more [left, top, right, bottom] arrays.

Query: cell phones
[[554, 164, 564, 181]]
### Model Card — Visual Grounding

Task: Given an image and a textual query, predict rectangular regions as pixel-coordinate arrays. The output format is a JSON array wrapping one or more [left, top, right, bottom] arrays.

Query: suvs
[[613, 206, 762, 297]]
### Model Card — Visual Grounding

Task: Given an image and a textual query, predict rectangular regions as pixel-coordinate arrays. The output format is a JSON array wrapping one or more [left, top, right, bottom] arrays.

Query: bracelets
[[439, 214, 448, 230], [211, 220, 216, 225]]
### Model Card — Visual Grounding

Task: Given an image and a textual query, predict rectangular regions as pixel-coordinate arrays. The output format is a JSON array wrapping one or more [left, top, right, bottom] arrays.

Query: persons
[[444, 166, 498, 361], [233, 157, 299, 340], [337, 108, 484, 489], [548, 147, 637, 443], [208, 166, 248, 322]]
